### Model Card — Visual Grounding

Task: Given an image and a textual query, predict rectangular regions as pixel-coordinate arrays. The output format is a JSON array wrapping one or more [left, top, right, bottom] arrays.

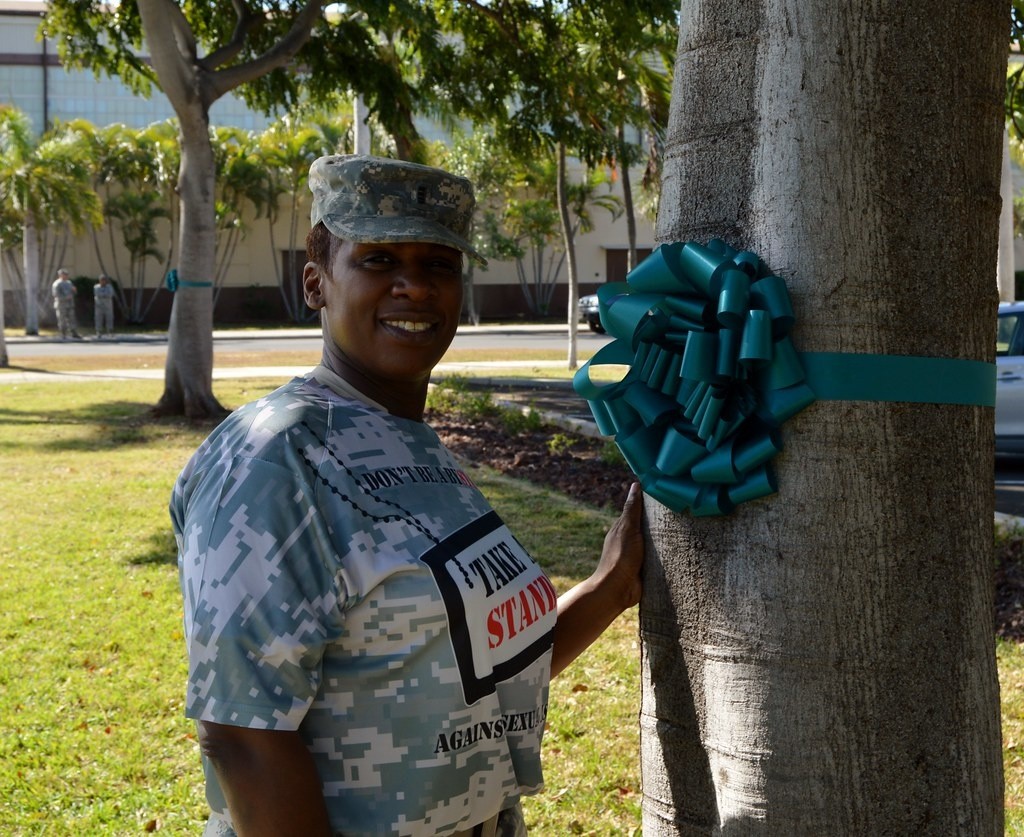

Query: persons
[[92, 273, 117, 338], [169, 154, 646, 837], [51, 268, 83, 340]]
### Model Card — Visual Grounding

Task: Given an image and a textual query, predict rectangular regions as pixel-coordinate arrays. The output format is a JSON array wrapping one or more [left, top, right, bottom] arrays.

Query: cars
[[991, 302, 1024, 468], [579, 292, 608, 335]]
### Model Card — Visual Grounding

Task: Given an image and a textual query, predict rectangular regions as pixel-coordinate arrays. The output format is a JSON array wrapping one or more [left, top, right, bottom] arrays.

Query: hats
[[308, 154, 488, 266], [58, 268, 69, 275]]
